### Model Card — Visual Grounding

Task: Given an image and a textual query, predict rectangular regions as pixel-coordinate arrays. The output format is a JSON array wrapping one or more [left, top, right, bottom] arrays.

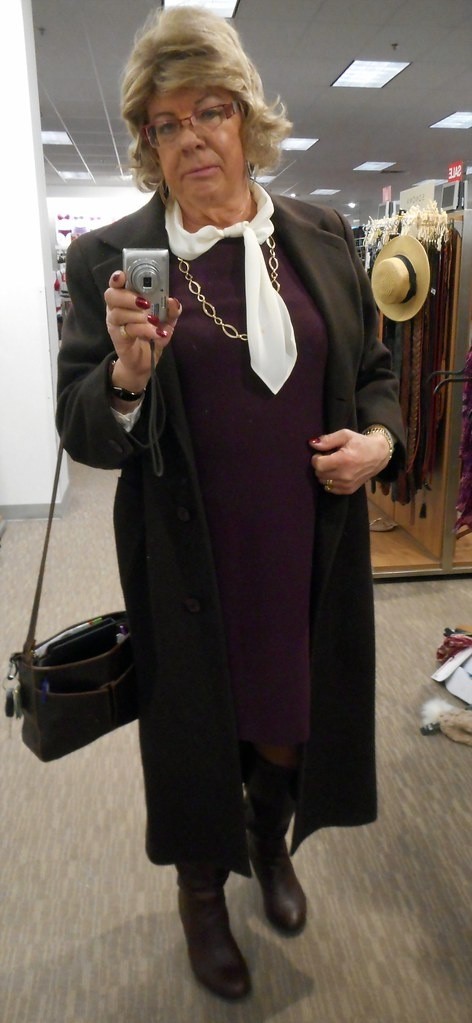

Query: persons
[[56, 262, 74, 319], [52, 6, 407, 1000], [56, 207, 72, 253]]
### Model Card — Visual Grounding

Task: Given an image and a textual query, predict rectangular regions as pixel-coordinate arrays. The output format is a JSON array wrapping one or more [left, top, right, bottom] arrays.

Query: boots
[[174, 862, 253, 999], [247, 748, 306, 936]]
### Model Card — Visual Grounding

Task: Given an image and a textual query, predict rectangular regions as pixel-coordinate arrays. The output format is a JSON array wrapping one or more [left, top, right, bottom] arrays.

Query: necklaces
[[177, 236, 281, 342]]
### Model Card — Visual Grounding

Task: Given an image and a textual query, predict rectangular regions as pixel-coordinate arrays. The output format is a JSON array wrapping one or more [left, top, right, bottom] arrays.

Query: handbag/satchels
[[11, 610, 140, 764]]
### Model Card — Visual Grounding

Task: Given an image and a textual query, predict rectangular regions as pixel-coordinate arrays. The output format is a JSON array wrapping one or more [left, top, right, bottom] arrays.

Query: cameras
[[123, 248, 170, 324]]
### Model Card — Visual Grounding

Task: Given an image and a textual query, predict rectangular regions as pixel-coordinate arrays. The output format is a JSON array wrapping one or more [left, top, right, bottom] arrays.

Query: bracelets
[[363, 426, 394, 462], [108, 359, 146, 402]]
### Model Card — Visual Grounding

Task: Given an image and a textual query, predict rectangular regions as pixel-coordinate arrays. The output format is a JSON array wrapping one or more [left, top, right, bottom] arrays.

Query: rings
[[325, 479, 332, 486], [325, 486, 330, 491], [120, 324, 132, 341]]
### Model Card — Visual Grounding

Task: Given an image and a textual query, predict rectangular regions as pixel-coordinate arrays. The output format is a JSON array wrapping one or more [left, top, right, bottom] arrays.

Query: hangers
[[427, 352, 472, 395]]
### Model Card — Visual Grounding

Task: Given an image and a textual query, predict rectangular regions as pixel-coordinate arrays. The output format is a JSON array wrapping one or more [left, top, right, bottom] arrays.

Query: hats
[[370, 234, 430, 321]]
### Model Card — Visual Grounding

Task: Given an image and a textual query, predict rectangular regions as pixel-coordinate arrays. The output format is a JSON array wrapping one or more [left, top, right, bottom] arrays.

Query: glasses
[[143, 102, 242, 148]]
